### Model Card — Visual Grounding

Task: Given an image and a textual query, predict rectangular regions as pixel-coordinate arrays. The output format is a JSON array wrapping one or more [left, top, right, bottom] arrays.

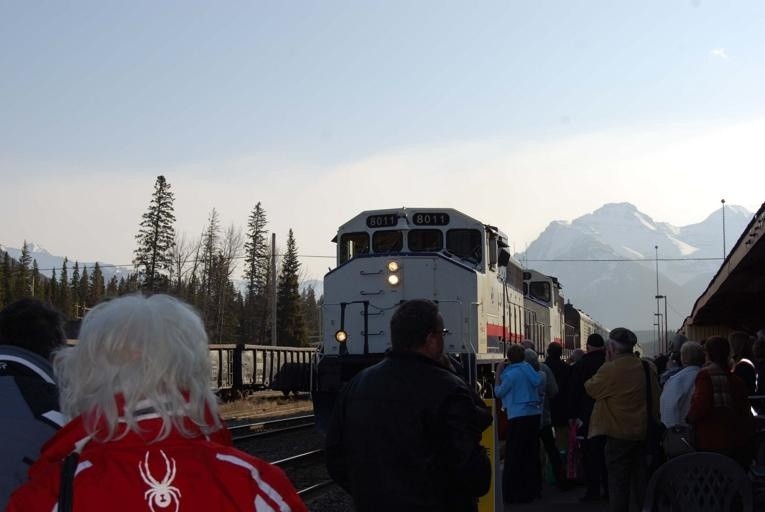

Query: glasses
[[431, 328, 449, 338]]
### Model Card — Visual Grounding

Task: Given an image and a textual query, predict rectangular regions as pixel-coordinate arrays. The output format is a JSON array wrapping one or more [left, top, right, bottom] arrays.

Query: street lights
[[653, 294, 668, 355]]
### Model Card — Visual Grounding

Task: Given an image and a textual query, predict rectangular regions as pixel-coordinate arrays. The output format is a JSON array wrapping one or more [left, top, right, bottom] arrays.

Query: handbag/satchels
[[661, 427, 697, 465]]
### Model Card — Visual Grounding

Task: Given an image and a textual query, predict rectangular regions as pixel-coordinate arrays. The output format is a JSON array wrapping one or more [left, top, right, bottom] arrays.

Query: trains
[[207, 343, 319, 404], [311, 206, 645, 440]]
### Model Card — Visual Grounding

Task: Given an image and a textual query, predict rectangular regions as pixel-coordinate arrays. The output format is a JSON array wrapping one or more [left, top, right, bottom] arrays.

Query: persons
[[427, 312, 494, 434], [323, 296, 492, 512], [489, 327, 764, 512], [0, 295, 78, 512], [0, 292, 309, 512]]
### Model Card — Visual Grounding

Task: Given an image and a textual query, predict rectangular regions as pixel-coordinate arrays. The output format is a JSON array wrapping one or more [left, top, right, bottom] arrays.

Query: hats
[[609, 328, 638, 346], [587, 333, 605, 347]]
